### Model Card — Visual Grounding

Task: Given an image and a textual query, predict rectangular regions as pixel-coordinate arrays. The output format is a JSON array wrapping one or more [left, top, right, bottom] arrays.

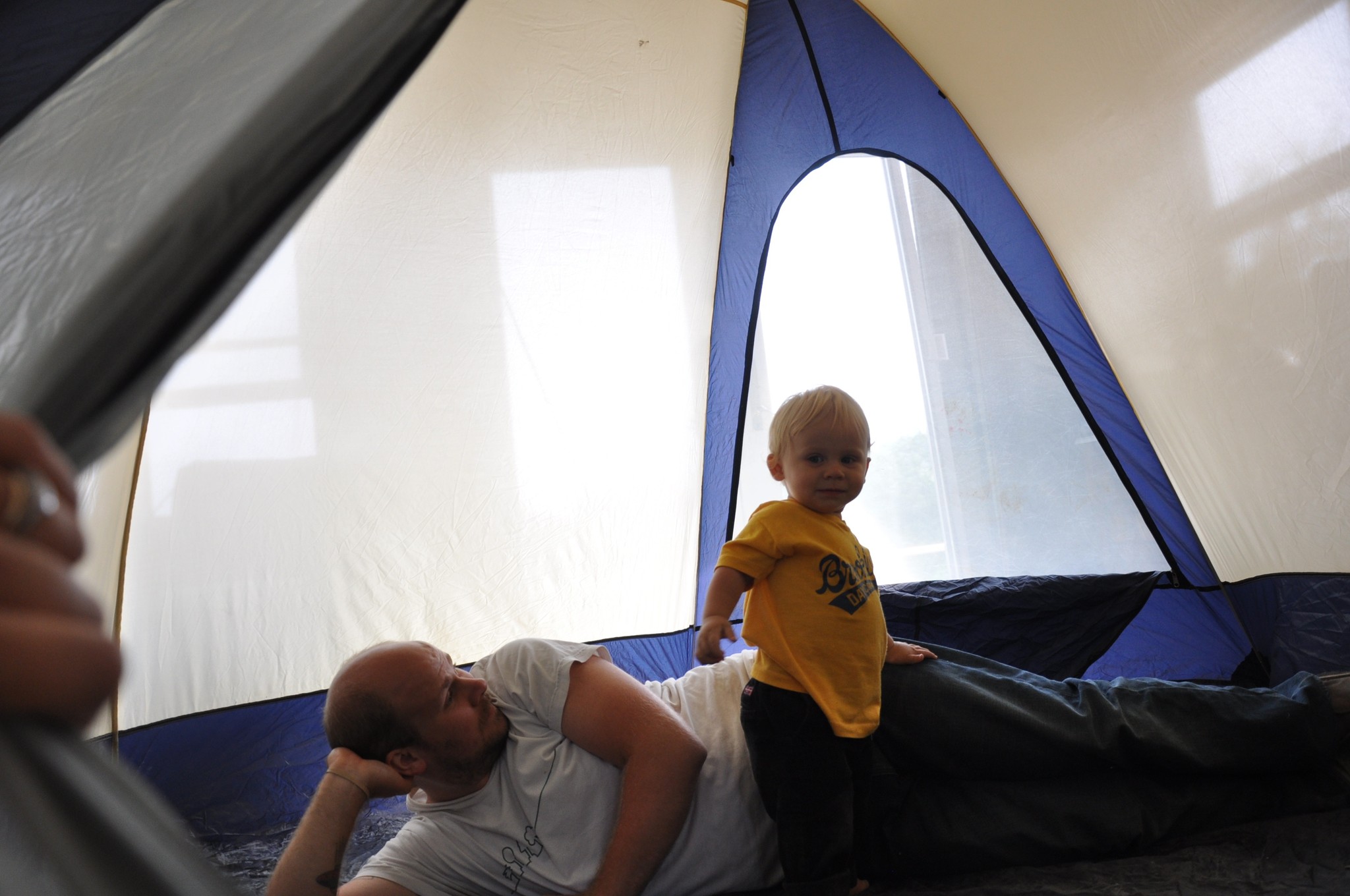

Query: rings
[[5, 468, 55, 536]]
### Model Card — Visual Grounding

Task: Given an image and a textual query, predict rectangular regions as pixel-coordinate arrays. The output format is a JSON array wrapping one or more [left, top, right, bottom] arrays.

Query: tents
[[0, 0, 1350, 896]]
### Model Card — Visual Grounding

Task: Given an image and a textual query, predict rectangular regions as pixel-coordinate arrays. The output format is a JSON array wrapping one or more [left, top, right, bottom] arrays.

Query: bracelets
[[327, 769, 370, 799]]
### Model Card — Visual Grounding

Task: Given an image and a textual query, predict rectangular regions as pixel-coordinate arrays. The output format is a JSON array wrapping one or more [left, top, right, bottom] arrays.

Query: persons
[[0, 404, 122, 731], [695, 385, 938, 894], [261, 640, 1350, 895]]
